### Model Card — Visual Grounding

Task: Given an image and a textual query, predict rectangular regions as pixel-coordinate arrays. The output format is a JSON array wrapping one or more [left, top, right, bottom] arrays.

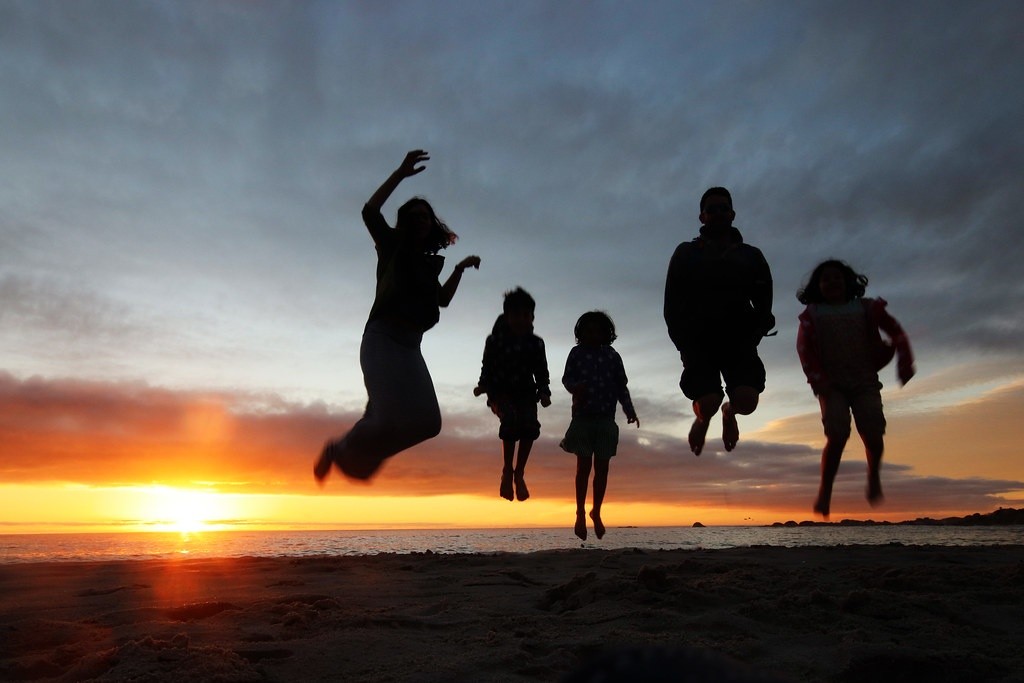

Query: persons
[[313, 149, 481, 481], [473, 285, 553, 502], [795, 259, 916, 516], [559, 310, 640, 541], [663, 187, 779, 456]]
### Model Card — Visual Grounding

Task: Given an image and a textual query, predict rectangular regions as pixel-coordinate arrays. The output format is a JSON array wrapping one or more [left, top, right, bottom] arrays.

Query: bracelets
[[455, 265, 464, 272]]
